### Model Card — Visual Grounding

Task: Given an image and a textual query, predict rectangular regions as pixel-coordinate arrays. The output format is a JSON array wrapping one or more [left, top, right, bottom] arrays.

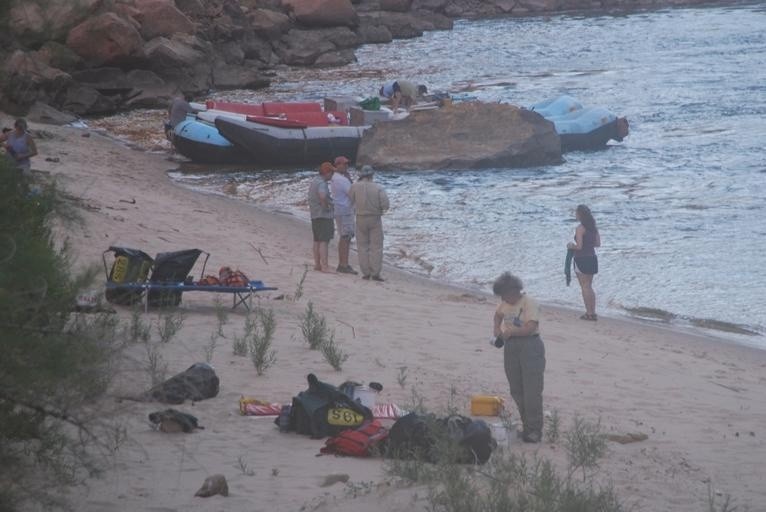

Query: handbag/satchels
[[390, 414, 496, 466], [320, 420, 390, 458], [276, 373, 374, 439]]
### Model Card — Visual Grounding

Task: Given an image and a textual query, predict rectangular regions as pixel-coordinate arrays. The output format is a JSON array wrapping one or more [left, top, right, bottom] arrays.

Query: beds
[[104, 279, 277, 313]]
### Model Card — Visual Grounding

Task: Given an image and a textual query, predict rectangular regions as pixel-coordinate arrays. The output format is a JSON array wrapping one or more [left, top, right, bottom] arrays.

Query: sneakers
[[336, 264, 358, 274], [580, 314, 597, 320], [362, 275, 384, 282], [517, 428, 542, 442]]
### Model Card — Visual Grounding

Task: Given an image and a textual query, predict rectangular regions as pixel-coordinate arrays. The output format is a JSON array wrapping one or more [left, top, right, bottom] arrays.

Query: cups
[[488, 336, 504, 349]]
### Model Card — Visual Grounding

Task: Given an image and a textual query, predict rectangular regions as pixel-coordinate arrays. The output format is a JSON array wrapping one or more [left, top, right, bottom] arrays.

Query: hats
[[334, 156, 352, 165], [319, 162, 337, 175], [358, 165, 374, 177]]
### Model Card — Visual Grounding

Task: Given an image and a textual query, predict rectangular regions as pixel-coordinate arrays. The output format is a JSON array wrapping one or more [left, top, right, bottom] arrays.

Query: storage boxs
[[471, 395, 503, 416]]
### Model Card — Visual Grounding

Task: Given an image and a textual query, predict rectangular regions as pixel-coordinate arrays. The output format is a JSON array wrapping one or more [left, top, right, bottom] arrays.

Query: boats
[[161, 92, 631, 168]]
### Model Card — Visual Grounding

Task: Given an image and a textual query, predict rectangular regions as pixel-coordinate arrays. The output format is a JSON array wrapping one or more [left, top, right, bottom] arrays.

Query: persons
[[0, 126, 12, 149], [3, 116, 39, 190], [379, 83, 394, 105], [564, 202, 601, 322], [390, 79, 428, 115], [488, 270, 546, 444], [347, 165, 391, 283], [163, 91, 202, 143], [330, 154, 359, 275], [306, 160, 338, 276]]
